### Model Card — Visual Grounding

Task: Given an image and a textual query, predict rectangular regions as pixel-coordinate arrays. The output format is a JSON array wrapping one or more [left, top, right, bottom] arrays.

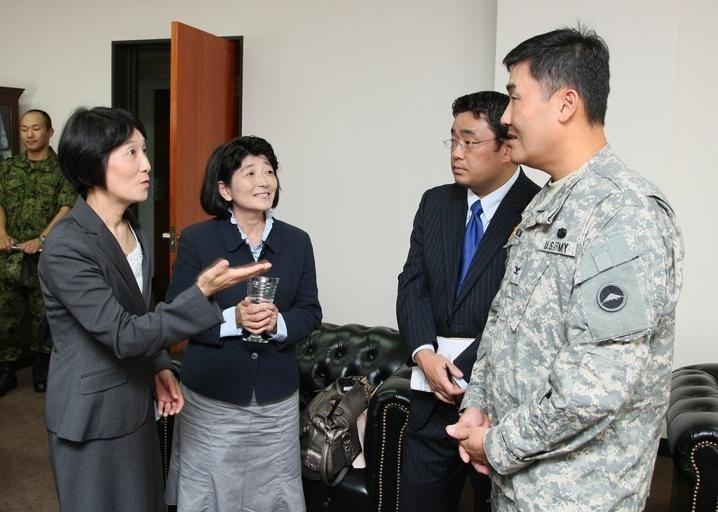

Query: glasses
[[442, 136, 498, 153]]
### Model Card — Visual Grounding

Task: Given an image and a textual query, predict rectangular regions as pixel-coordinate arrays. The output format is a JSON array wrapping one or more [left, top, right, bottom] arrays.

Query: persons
[[396, 90, 549, 511], [445, 24, 683, 511], [45, 104, 272, 510], [166, 132, 322, 511], [1, 110, 77, 396]]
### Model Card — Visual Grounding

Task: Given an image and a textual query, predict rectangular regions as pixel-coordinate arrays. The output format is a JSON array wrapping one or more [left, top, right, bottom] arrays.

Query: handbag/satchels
[[298, 375, 373, 489]]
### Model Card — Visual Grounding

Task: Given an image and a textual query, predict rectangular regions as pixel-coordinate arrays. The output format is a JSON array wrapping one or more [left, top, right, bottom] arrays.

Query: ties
[[454, 200, 483, 297]]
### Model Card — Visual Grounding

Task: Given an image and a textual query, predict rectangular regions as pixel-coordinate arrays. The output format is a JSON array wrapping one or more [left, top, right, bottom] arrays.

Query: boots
[[0, 361, 17, 397], [32, 352, 49, 393]]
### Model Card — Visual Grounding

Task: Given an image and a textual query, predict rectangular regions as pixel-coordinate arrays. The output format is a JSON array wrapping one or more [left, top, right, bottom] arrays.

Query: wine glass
[[240, 275, 279, 345]]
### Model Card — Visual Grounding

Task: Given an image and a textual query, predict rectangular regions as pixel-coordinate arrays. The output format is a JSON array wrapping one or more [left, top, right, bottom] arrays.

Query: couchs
[[667, 360, 716, 510], [157, 319, 424, 509]]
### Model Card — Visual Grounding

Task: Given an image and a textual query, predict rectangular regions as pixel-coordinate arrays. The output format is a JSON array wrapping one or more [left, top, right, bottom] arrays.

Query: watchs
[[37, 232, 47, 245]]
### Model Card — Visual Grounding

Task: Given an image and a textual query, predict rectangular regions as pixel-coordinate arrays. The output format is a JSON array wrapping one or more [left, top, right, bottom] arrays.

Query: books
[[410, 335, 477, 396], [9, 245, 43, 253]]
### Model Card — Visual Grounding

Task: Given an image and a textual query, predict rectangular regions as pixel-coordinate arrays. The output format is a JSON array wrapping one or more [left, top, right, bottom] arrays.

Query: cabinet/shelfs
[[0, 86, 24, 176]]
